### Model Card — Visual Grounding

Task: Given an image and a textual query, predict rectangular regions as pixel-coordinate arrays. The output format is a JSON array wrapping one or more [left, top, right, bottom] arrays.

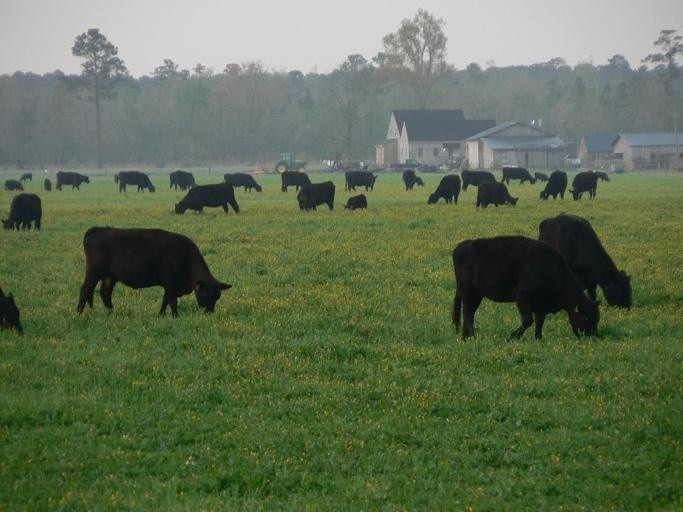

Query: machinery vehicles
[[275, 153, 307, 175]]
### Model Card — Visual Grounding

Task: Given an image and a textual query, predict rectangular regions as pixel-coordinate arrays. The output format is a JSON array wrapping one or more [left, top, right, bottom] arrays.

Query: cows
[[162, 168, 379, 215], [0, 281, 24, 336], [451, 233, 602, 341], [537, 214, 634, 311], [398, 166, 609, 210], [76, 224, 233, 317], [43, 169, 91, 191], [116, 168, 155, 194], [2, 172, 43, 231]]
